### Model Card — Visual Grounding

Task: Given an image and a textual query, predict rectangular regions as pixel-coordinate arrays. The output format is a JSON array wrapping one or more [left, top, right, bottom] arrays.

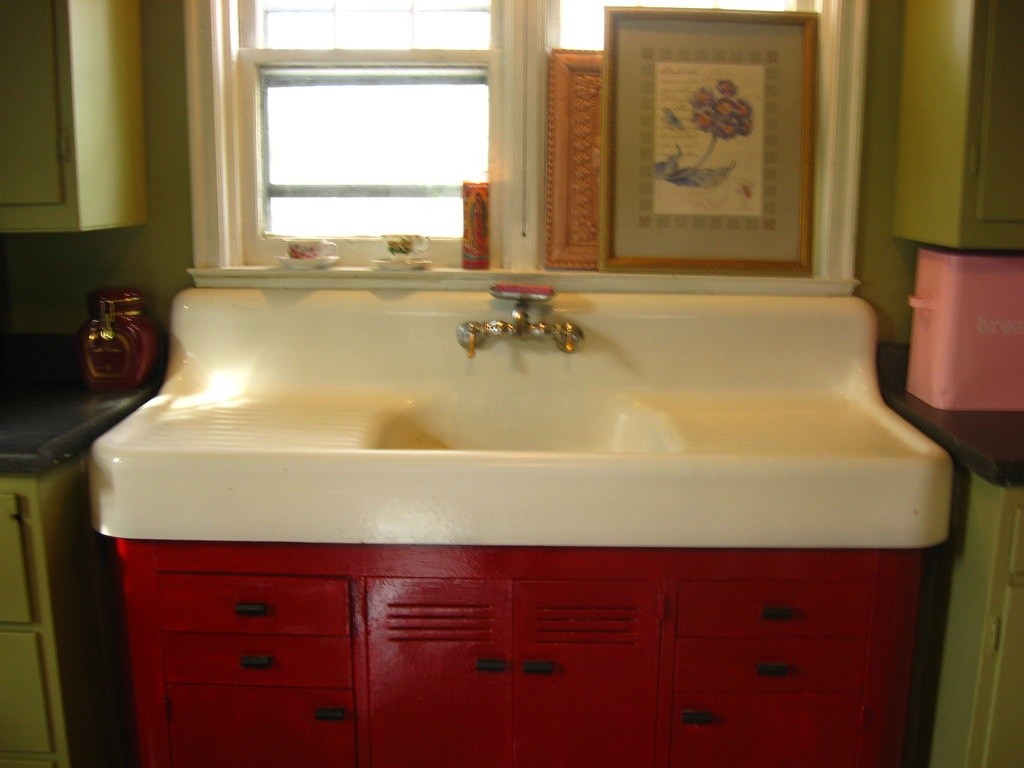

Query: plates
[[372, 261, 433, 271], [278, 257, 341, 268]]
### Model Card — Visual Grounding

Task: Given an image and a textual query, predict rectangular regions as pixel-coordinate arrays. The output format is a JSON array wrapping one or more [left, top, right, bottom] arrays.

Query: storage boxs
[[904, 245, 1024, 413]]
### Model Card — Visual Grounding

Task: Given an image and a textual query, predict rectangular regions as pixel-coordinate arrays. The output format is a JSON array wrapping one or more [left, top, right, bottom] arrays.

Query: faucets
[[455, 285, 585, 360]]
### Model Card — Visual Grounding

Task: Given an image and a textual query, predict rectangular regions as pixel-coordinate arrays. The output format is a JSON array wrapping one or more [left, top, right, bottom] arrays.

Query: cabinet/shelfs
[[893, 1, 1024, 254]]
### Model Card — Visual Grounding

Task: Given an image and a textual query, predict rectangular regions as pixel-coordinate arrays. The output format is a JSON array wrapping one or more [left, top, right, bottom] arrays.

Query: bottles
[[73, 287, 159, 388]]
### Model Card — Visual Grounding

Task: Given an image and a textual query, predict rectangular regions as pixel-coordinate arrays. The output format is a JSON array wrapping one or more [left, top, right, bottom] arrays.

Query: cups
[[381, 235, 431, 261], [284, 237, 336, 259]]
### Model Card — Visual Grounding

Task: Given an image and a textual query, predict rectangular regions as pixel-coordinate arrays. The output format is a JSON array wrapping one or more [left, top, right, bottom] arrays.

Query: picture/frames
[[0, 454, 1024, 768], [597, 5, 818, 279]]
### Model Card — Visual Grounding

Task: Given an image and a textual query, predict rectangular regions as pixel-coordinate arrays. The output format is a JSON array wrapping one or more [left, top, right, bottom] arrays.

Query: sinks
[[358, 388, 689, 459]]
